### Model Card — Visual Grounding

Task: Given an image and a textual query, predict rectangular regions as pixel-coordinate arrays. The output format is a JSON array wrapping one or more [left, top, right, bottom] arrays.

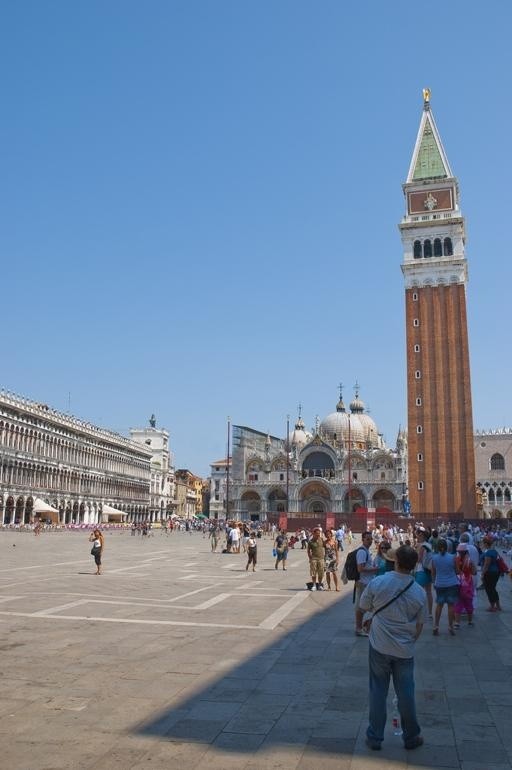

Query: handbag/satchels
[[362, 615, 373, 633], [90, 546, 101, 555], [306, 582, 323, 589], [497, 557, 508, 572], [277, 545, 285, 554]]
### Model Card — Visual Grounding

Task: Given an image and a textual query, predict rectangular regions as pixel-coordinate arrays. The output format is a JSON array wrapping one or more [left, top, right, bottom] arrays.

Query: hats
[[382, 549, 396, 561], [456, 543, 469, 552]]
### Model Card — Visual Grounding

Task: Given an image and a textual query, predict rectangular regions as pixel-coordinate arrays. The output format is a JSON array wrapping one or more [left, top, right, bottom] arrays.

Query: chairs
[[0, 511, 132, 534]]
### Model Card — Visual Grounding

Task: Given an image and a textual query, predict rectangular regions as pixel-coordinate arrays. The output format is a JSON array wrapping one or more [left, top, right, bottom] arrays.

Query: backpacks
[[448, 538, 460, 554], [419, 545, 433, 574], [345, 547, 368, 581]]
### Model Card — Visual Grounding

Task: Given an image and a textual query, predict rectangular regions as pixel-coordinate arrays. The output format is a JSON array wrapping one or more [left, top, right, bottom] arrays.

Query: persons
[[130, 519, 154, 539], [89, 529, 103, 575], [357, 545, 430, 750], [19, 518, 53, 536], [353, 521, 512, 635], [160, 517, 353, 591]]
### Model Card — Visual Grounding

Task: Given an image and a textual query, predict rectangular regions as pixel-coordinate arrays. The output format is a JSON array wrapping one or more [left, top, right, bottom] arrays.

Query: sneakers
[[310, 587, 317, 591], [318, 586, 326, 591], [355, 628, 368, 637], [404, 735, 423, 749], [485, 606, 501, 612], [433, 622, 474, 636], [365, 737, 381, 750]]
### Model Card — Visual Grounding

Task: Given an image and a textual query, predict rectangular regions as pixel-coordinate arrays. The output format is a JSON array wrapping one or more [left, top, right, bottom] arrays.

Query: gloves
[[273, 549, 277, 556]]
